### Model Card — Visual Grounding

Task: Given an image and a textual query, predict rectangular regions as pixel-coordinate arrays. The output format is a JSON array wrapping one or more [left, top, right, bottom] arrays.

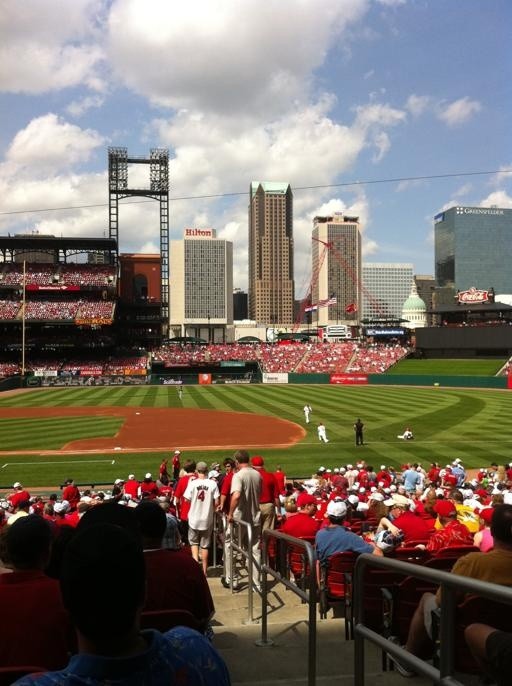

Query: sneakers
[[385, 636, 416, 677]]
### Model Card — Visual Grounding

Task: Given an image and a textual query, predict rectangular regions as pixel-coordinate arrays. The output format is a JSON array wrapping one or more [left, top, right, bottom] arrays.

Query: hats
[[326, 469, 331, 473], [333, 467, 339, 472], [328, 502, 347, 517], [383, 495, 410, 507], [446, 464, 452, 468], [318, 466, 326, 472], [13, 482, 22, 488], [340, 467, 346, 475], [390, 485, 396, 491], [417, 466, 422, 472], [433, 500, 458, 518], [479, 508, 494, 524], [122, 493, 132, 500], [368, 493, 384, 501], [174, 450, 181, 454], [371, 487, 377, 492], [378, 482, 384, 487], [347, 464, 353, 469], [62, 500, 70, 511], [1, 502, 9, 509], [400, 486, 406, 490], [335, 485, 343, 492], [296, 493, 323, 507], [357, 464, 364, 468], [54, 503, 66, 513], [347, 495, 359, 504], [196, 462, 209, 471], [381, 465, 386, 470], [474, 490, 487, 498], [115, 479, 124, 485], [471, 494, 481, 500], [251, 455, 264, 467], [456, 458, 461, 463], [452, 461, 458, 466], [135, 499, 167, 537], [145, 473, 152, 478], [128, 474, 135, 480], [355, 482, 360, 487], [59, 502, 147, 611]]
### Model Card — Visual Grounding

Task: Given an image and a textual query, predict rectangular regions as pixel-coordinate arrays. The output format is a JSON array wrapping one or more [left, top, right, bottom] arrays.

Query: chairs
[[392, 547, 431, 566], [380, 574, 440, 671], [429, 624, 431, 624], [0, 577, 68, 665], [319, 552, 361, 619], [430, 580, 511, 668], [285, 536, 317, 593], [262, 529, 284, 572], [403, 540, 429, 548], [437, 545, 480, 558], [421, 557, 459, 573], [0, 666, 48, 686], [345, 509, 380, 537], [137, 610, 196, 634]]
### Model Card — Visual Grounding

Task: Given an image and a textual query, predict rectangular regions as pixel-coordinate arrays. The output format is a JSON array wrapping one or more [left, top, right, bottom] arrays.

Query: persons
[[397, 427, 413, 440], [182, 461, 220, 577], [272, 461, 289, 521], [158, 457, 169, 486], [382, 493, 437, 552], [172, 448, 181, 483], [0, 471, 183, 550], [218, 457, 236, 530], [464, 623, 512, 686], [0, 300, 116, 323], [0, 264, 120, 287], [279, 457, 511, 513], [208, 462, 223, 491], [176, 385, 185, 401], [507, 359, 512, 390], [317, 422, 331, 443], [441, 316, 512, 328], [175, 460, 199, 549], [6, 521, 234, 686], [130, 499, 215, 629], [1, 355, 150, 381], [314, 501, 384, 570], [257, 343, 409, 375], [303, 404, 313, 425], [425, 500, 475, 551], [152, 343, 257, 361], [277, 493, 320, 554], [0, 515, 66, 650], [248, 455, 274, 568], [373, 517, 406, 551], [221, 448, 261, 589], [354, 419, 365, 447], [385, 505, 512, 679], [472, 507, 498, 553]]
